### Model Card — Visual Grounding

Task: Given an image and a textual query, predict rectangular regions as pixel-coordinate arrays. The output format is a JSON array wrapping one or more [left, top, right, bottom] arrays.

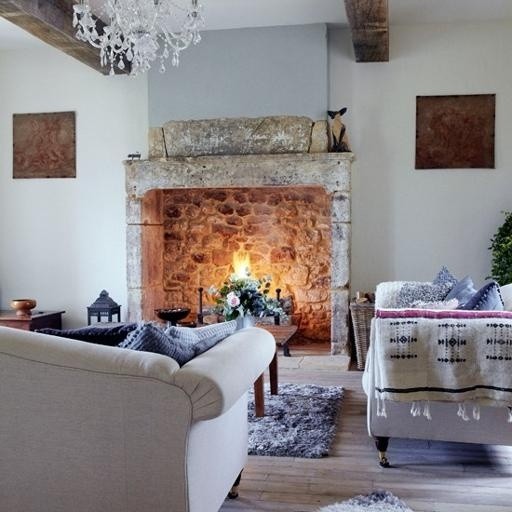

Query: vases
[[243, 309, 254, 326]]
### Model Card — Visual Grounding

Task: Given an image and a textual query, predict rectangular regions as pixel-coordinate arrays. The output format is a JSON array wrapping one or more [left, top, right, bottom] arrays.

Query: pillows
[[397, 263, 505, 311], [33, 320, 238, 367]]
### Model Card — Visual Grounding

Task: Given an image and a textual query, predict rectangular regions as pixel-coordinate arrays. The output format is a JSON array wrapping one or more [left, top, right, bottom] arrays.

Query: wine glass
[[11, 298, 37, 317]]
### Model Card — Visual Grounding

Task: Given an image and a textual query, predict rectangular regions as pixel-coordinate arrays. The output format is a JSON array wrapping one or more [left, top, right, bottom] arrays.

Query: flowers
[[209, 269, 290, 324]]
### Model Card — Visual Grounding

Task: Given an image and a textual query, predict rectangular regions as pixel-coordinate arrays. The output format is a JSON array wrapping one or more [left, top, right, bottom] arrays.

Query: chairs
[[361, 281, 512, 469], [1, 326, 278, 512]]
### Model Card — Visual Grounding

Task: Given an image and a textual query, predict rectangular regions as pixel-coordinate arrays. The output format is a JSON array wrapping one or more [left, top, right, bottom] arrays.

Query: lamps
[[72, 0, 207, 80]]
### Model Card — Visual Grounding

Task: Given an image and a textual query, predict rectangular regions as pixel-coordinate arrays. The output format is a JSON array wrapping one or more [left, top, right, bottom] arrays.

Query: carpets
[[317, 489, 415, 512], [246, 382, 345, 459]]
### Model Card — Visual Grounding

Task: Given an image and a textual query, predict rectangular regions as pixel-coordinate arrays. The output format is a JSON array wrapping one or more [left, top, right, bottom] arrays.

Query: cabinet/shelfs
[[0, 309, 66, 331]]
